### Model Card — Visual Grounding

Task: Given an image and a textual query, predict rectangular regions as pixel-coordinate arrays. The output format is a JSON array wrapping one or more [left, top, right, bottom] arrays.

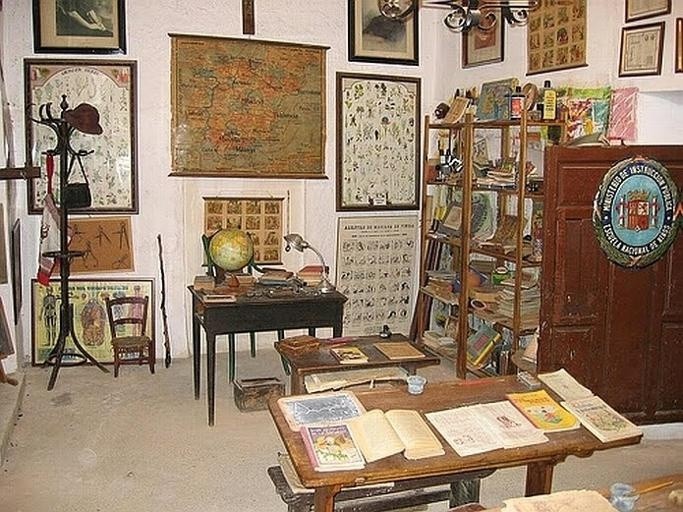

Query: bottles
[[534, 79, 557, 122], [436, 148, 456, 184], [510, 86, 526, 121]]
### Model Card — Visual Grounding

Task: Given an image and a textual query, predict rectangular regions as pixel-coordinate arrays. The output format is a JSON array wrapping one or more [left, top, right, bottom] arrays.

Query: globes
[[210, 227, 255, 294]]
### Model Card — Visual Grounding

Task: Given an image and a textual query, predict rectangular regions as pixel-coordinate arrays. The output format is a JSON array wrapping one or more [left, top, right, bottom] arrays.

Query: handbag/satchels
[[62, 153, 91, 209]]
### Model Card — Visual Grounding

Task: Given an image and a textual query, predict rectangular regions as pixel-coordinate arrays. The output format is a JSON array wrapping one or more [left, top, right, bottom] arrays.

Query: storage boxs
[[232, 375, 289, 416]]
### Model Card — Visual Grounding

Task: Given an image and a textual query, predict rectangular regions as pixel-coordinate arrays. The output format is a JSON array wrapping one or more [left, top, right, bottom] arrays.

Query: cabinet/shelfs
[[405, 105, 571, 381]]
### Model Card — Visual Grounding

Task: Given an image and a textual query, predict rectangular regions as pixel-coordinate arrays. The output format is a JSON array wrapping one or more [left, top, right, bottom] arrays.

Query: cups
[[405, 376, 426, 394], [608, 482, 640, 511]]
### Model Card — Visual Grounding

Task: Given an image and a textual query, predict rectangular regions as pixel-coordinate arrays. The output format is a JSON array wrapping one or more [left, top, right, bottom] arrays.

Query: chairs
[[101, 294, 158, 380], [197, 229, 316, 384]]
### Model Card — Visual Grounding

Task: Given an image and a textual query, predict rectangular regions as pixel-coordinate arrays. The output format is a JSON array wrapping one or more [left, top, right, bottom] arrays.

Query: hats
[[64, 102, 103, 135]]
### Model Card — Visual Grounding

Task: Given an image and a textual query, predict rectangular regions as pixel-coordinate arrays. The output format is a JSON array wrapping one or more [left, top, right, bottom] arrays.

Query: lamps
[[282, 232, 339, 297], [375, 0, 545, 33]]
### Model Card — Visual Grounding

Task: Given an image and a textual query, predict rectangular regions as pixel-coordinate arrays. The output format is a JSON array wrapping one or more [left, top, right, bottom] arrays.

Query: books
[[501, 486, 618, 512], [276, 365, 644, 471], [191, 263, 331, 306], [419, 78, 637, 365]]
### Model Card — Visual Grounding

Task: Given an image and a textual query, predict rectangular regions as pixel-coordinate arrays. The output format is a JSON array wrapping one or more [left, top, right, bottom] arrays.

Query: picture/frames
[[458, 1, 507, 71], [9, 217, 23, 327], [30, 1, 128, 57], [624, 1, 672, 25], [616, 19, 668, 80], [673, 17, 683, 75], [29, 278, 156, 370], [20, 56, 140, 217], [345, 0, 423, 70], [332, 69, 425, 213]]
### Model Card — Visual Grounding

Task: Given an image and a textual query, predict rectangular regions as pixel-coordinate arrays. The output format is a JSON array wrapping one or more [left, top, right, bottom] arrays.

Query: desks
[[492, 470, 683, 510], [264, 366, 646, 510], [185, 279, 346, 431], [268, 331, 440, 398]]
